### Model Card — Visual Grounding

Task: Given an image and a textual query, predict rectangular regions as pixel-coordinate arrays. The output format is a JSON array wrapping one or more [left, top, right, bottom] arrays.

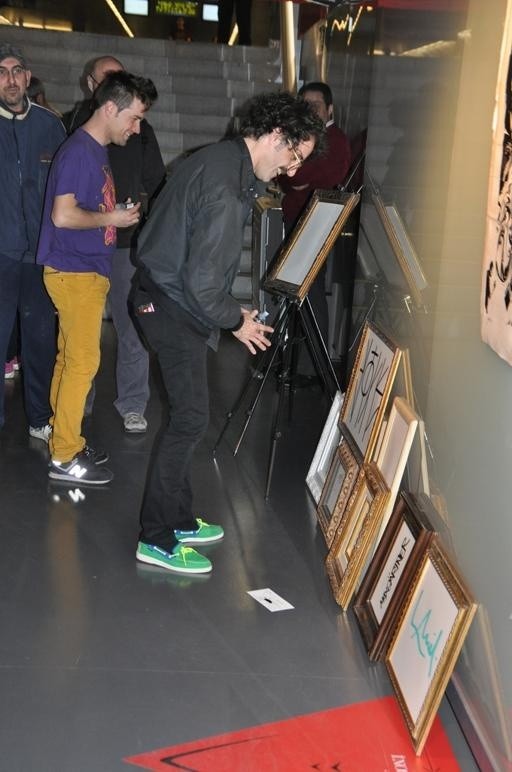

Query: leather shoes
[[278, 368, 321, 388]]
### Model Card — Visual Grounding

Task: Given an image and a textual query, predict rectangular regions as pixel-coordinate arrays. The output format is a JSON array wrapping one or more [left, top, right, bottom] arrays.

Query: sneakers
[[136, 541, 213, 573], [4, 354, 148, 485], [173, 517, 224, 543]]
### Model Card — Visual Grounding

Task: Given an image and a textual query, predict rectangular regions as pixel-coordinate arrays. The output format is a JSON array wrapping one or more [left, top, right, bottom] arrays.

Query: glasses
[[286, 138, 304, 171]]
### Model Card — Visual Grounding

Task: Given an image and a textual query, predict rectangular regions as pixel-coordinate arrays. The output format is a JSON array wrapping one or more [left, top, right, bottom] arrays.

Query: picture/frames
[[385, 539, 477, 755], [352, 492, 436, 662], [317, 440, 359, 551], [337, 320, 402, 470], [356, 395, 417, 593], [395, 347, 460, 562], [325, 462, 392, 610], [306, 394, 343, 505], [258, 188, 362, 301], [363, 185, 428, 311]]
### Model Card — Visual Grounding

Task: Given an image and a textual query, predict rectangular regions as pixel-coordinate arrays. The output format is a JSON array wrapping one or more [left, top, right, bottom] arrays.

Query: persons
[[0, 54, 67, 445], [35, 69, 159, 487], [0, 76, 61, 379], [128, 89, 329, 577], [59, 55, 168, 434], [279, 81, 354, 392]]
